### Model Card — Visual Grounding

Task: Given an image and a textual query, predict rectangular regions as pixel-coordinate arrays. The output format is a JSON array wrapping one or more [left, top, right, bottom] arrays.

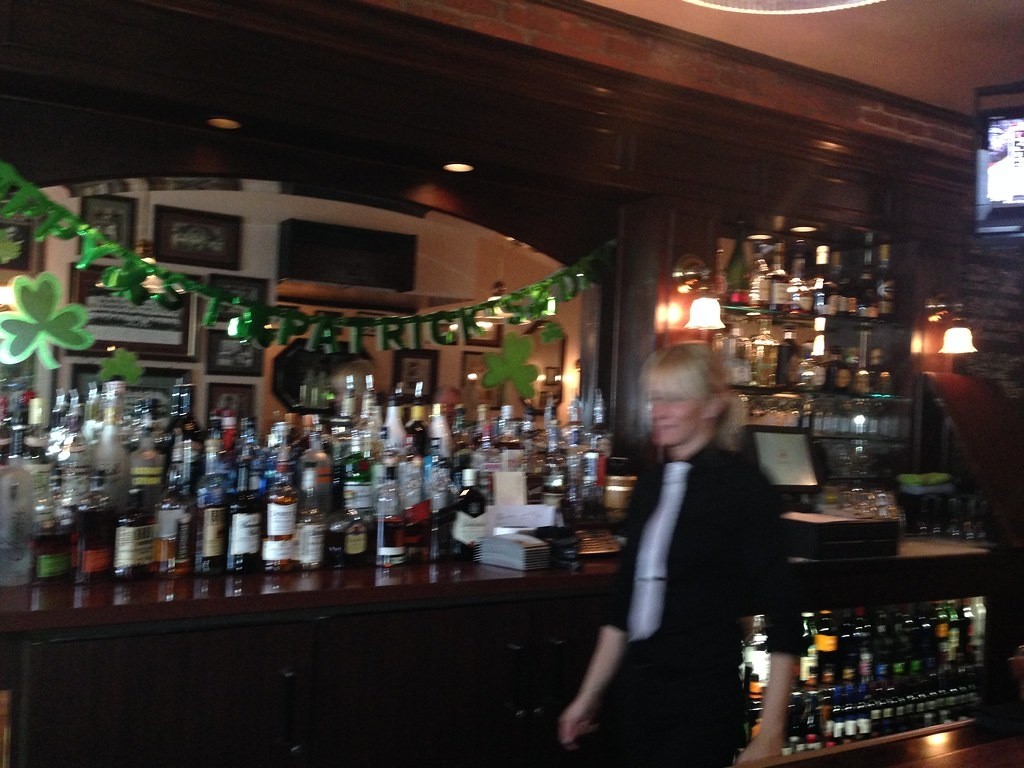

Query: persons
[[557, 345, 809, 768]]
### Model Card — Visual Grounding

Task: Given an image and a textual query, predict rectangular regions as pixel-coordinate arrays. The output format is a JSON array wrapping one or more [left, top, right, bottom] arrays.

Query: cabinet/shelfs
[[0, 534, 994, 768], [714, 219, 913, 482]]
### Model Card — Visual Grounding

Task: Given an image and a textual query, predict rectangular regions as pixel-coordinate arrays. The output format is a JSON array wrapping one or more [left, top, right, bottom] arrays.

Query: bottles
[[739, 598, 987, 759], [713, 217, 991, 540], [0, 372, 641, 585]]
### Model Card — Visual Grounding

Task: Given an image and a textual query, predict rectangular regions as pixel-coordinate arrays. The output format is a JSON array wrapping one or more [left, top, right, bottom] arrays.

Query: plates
[[475, 535, 554, 572]]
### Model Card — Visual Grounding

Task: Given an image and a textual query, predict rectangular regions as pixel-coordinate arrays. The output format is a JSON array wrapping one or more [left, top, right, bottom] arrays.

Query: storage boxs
[[783, 511, 901, 558], [277, 217, 418, 293]]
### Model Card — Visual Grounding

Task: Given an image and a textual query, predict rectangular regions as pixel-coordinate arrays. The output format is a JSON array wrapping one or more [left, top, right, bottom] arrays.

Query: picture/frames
[[464, 323, 502, 348], [206, 381, 256, 428], [153, 202, 243, 269], [745, 423, 821, 494], [393, 347, 438, 407], [204, 328, 264, 378], [545, 366, 558, 386], [0, 214, 45, 285], [209, 272, 271, 323], [77, 194, 138, 260], [64, 261, 204, 363], [462, 348, 504, 412], [271, 338, 380, 417], [68, 361, 191, 417]]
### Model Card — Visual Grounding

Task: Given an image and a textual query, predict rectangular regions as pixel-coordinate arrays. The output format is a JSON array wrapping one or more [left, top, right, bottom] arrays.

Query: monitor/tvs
[[741, 423, 819, 492], [984, 113, 1024, 210]]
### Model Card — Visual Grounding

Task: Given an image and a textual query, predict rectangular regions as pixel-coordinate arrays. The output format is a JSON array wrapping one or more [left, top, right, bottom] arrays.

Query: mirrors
[[0, 177, 597, 448], [519, 320, 567, 414]]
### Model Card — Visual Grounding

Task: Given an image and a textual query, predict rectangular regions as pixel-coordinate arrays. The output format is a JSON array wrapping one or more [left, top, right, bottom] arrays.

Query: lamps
[[670, 254, 725, 332], [923, 292, 978, 356], [476, 281, 514, 321]]
